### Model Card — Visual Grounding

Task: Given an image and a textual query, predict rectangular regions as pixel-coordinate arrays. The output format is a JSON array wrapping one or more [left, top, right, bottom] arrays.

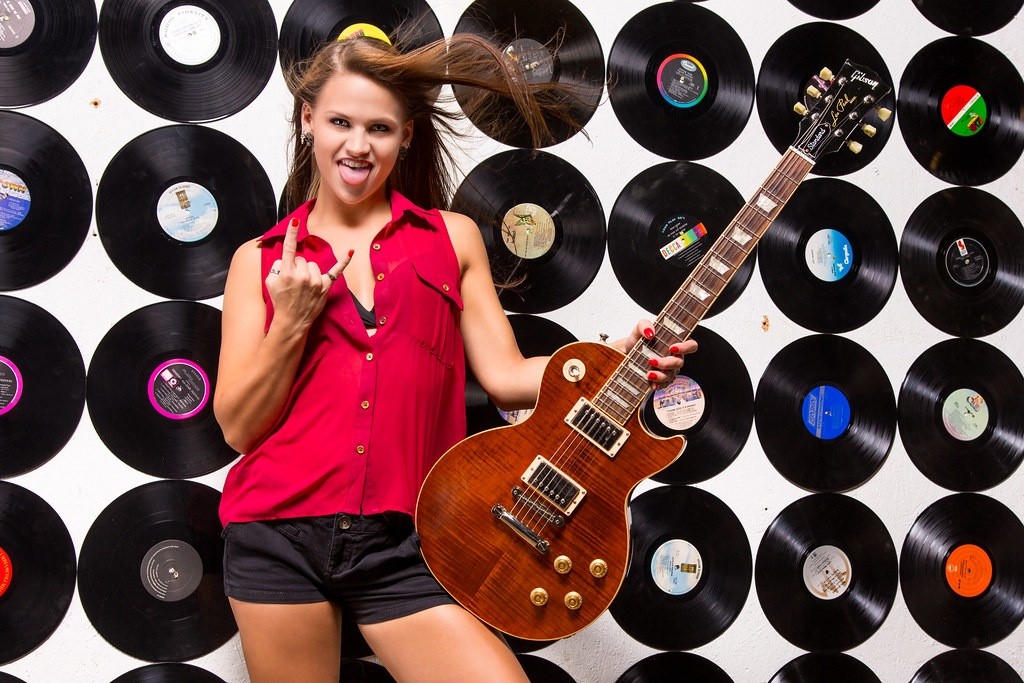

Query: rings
[[326, 271, 336, 282], [268, 268, 280, 274]]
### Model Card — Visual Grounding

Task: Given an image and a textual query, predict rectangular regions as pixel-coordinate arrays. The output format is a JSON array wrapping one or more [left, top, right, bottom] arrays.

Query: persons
[[212, 32, 698, 683]]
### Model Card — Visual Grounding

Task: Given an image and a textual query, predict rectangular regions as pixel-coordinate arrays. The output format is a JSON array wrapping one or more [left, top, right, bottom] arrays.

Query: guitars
[[414, 63, 894, 642]]
